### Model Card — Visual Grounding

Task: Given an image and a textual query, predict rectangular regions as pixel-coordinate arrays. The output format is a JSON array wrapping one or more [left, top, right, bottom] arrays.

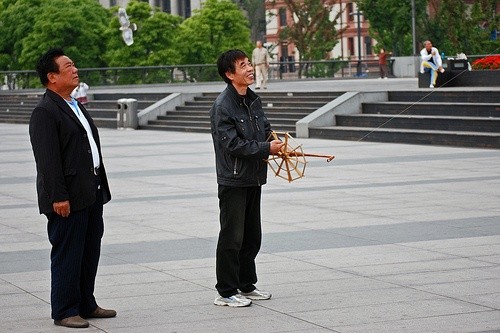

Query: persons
[[72, 81, 89, 108], [376, 48, 389, 79], [420, 39, 445, 88], [210, 49, 285, 307], [28, 48, 117, 328], [251, 40, 271, 88]]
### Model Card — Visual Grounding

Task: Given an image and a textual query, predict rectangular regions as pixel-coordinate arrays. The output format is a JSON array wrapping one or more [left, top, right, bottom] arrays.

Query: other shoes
[[430, 84, 435, 89], [54, 314, 89, 328], [440, 68, 445, 74], [79, 306, 116, 318]]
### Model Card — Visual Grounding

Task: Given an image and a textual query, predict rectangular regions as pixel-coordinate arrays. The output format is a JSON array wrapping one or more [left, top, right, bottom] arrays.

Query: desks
[[442, 60, 470, 70]]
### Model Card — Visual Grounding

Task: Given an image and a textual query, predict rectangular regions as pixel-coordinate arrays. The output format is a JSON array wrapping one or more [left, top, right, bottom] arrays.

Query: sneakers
[[237, 288, 272, 300], [214, 295, 254, 307]]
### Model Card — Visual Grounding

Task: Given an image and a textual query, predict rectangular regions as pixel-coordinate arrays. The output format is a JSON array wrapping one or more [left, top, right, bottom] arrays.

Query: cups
[[441, 52, 445, 59]]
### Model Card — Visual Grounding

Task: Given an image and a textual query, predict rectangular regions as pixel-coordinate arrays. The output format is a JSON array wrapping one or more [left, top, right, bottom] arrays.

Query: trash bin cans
[[116, 98, 137, 130], [446, 58, 469, 71]]
